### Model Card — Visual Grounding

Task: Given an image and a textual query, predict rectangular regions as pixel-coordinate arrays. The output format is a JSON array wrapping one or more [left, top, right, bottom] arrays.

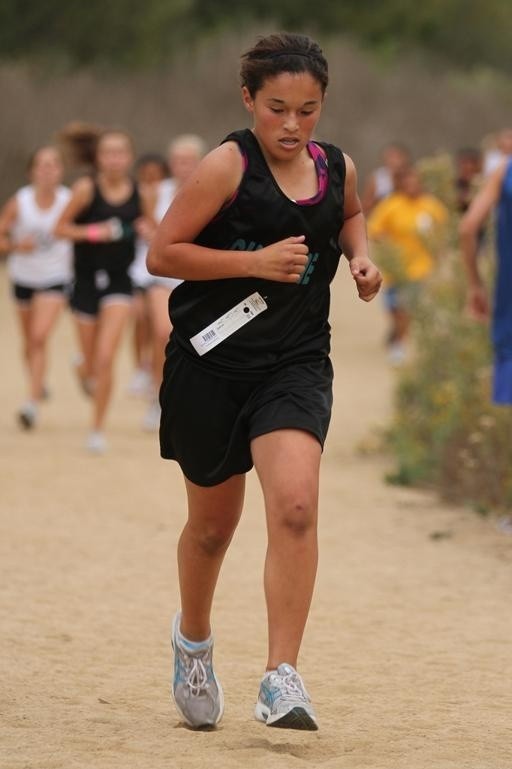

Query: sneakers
[[87, 431, 107, 450], [20, 402, 43, 427], [170, 611, 224, 731], [255, 658, 318, 730]]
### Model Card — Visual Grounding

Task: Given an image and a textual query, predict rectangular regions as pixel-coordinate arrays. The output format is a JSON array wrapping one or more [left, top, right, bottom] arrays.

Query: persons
[[128, 136, 205, 425], [453, 126, 511, 411], [360, 144, 449, 362], [55, 122, 158, 450], [145, 30, 385, 736], [2, 141, 75, 430]]
